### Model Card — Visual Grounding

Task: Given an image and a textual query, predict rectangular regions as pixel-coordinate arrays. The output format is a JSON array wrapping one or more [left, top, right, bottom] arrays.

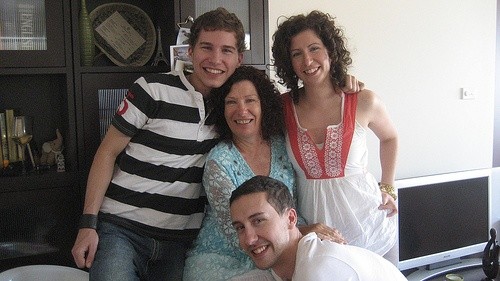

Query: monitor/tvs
[[392, 167, 500, 281]]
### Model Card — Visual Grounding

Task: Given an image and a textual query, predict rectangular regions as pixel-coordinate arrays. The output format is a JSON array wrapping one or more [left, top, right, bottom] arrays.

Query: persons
[[266, 11, 398, 258], [183, 66, 348, 281], [72, 7, 365, 281], [229, 176, 409, 281]]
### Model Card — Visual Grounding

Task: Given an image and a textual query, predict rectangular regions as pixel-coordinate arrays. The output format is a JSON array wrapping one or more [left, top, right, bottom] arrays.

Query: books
[[0, 109, 26, 167]]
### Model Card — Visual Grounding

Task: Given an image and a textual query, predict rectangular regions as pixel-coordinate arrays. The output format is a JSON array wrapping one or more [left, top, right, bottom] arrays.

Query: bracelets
[[79, 214, 98, 229], [378, 182, 398, 200]]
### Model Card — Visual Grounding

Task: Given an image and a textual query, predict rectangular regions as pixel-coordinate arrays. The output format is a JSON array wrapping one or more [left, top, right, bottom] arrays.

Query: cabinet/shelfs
[[0, 0, 271, 272]]
